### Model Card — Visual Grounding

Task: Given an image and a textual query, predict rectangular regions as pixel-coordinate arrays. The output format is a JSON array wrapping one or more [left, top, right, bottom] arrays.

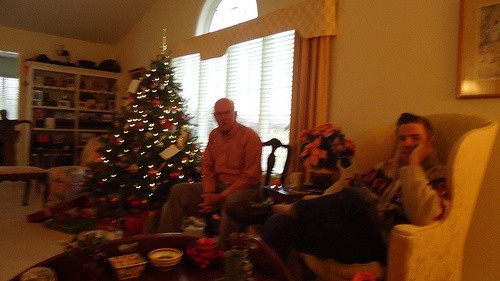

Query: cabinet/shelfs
[[23, 60, 121, 169]]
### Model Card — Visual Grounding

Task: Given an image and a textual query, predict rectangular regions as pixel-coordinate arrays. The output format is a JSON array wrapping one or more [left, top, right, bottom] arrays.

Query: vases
[[310, 164, 343, 192]]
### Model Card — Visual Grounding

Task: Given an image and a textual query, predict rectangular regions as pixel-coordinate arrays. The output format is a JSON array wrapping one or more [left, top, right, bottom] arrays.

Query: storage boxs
[[31, 89, 44, 102], [44, 117, 56, 129], [36, 120, 45, 128], [100, 114, 113, 122], [65, 78, 75, 89], [106, 99, 116, 110], [59, 99, 71, 108], [34, 132, 50, 142]]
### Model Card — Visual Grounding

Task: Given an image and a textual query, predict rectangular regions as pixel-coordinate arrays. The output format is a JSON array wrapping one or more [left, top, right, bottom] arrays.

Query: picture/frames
[[455, 0, 500, 100]]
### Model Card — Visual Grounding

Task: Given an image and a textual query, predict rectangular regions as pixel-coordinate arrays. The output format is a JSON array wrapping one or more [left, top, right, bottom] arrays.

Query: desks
[[9, 233, 286, 281]]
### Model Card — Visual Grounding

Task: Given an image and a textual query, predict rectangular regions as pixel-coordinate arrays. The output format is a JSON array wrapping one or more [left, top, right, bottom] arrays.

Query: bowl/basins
[[147, 248, 184, 267], [107, 252, 148, 280], [20, 266, 57, 281]]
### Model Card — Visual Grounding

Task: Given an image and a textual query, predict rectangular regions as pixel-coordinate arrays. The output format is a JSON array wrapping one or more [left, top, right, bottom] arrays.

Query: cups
[[222, 249, 248, 281]]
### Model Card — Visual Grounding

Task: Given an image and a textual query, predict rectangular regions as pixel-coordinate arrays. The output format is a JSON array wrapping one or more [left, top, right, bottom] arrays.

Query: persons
[[262, 113, 449, 276], [155, 99, 262, 237]]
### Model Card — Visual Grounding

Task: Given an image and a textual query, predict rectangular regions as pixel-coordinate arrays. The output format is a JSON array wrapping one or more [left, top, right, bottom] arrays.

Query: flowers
[[294, 122, 357, 170], [57, 232, 107, 269]]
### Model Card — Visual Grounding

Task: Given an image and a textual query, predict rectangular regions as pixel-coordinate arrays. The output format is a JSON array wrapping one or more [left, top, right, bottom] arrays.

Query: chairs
[[0, 118, 51, 210], [232, 138, 293, 234], [303, 112, 500, 281], [45, 135, 105, 200]]
